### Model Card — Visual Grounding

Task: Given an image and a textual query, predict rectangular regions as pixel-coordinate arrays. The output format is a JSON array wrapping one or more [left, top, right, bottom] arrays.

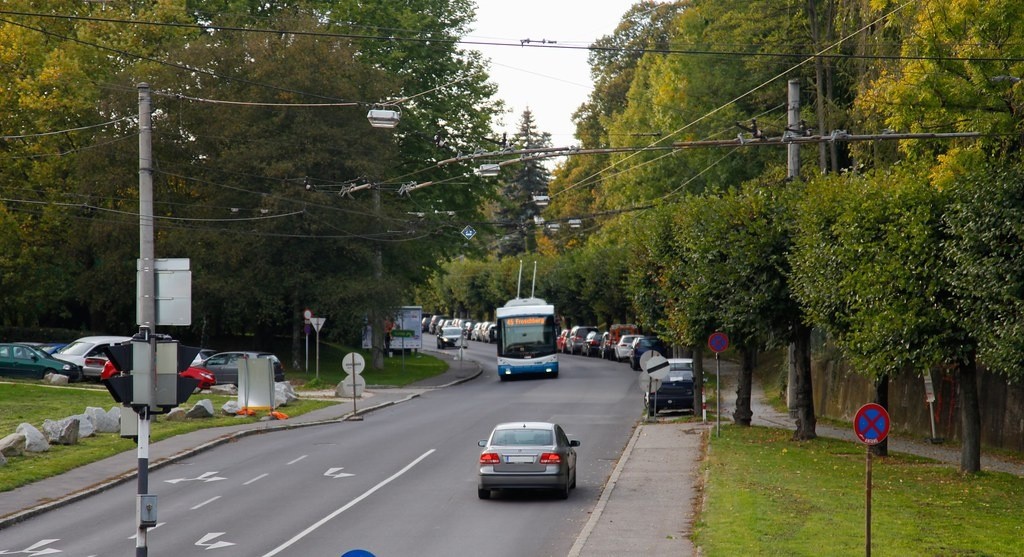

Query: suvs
[[565, 325, 600, 355], [606, 324, 641, 358]]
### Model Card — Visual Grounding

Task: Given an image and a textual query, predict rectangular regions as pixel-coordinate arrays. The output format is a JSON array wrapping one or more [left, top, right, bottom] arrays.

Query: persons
[[384, 317, 396, 356]]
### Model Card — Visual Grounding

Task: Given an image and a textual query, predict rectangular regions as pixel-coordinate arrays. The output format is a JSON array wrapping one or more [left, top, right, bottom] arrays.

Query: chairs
[[534, 433, 548, 443], [502, 435, 517, 443]]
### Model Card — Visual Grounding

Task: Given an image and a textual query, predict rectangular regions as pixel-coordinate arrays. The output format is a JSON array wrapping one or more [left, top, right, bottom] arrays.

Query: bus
[[490, 296, 562, 381]]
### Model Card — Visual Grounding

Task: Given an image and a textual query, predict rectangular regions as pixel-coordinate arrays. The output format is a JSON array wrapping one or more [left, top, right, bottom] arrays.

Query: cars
[[421, 312, 498, 343], [100, 359, 215, 395], [193, 351, 285, 386], [478, 421, 580, 499], [599, 333, 610, 358], [644, 357, 695, 408], [436, 325, 468, 349], [614, 334, 645, 362], [649, 372, 695, 415], [581, 331, 606, 356], [1, 344, 81, 386], [629, 337, 667, 371], [52, 335, 132, 378], [83, 354, 112, 377], [557, 329, 572, 352], [3, 343, 65, 357]]
